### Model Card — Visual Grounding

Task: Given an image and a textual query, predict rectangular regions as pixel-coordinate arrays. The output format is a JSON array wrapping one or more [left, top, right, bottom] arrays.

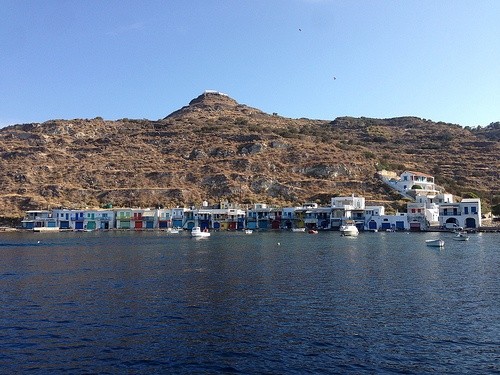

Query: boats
[[292, 227, 306, 233], [192, 230, 211, 237], [426, 239, 445, 247], [453, 233, 470, 241], [168, 227, 179, 233], [340, 225, 359, 236]]
